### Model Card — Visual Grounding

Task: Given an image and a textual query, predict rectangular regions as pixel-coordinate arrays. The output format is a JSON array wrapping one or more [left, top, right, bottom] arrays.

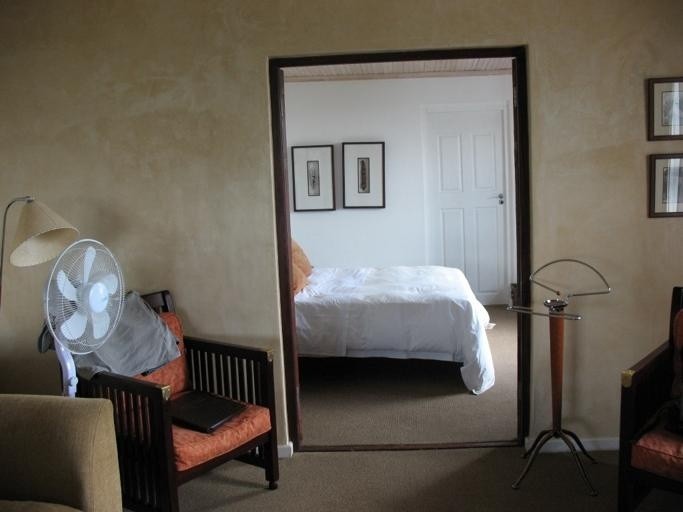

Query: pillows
[[292, 261, 308, 294], [292, 235, 314, 276]]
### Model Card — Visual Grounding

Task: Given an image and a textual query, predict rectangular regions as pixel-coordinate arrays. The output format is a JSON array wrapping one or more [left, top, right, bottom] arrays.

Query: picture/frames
[[644, 75, 682, 142], [292, 144, 335, 213], [342, 140, 387, 207], [646, 151, 682, 218]]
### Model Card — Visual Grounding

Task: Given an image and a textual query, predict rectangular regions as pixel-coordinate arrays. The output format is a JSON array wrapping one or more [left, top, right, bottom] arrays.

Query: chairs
[[0, 392, 123, 512], [62, 287, 279, 512], [618, 285, 683, 512]]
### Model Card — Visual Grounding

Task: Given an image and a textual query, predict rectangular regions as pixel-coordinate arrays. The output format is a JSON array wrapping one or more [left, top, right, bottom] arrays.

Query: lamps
[[0, 193, 80, 294]]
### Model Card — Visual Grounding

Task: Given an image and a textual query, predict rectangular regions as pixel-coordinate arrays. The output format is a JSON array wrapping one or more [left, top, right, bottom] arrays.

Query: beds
[[287, 264, 496, 396]]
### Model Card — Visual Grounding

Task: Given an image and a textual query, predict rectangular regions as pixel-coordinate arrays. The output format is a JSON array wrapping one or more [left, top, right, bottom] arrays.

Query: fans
[[42, 238, 126, 395]]
